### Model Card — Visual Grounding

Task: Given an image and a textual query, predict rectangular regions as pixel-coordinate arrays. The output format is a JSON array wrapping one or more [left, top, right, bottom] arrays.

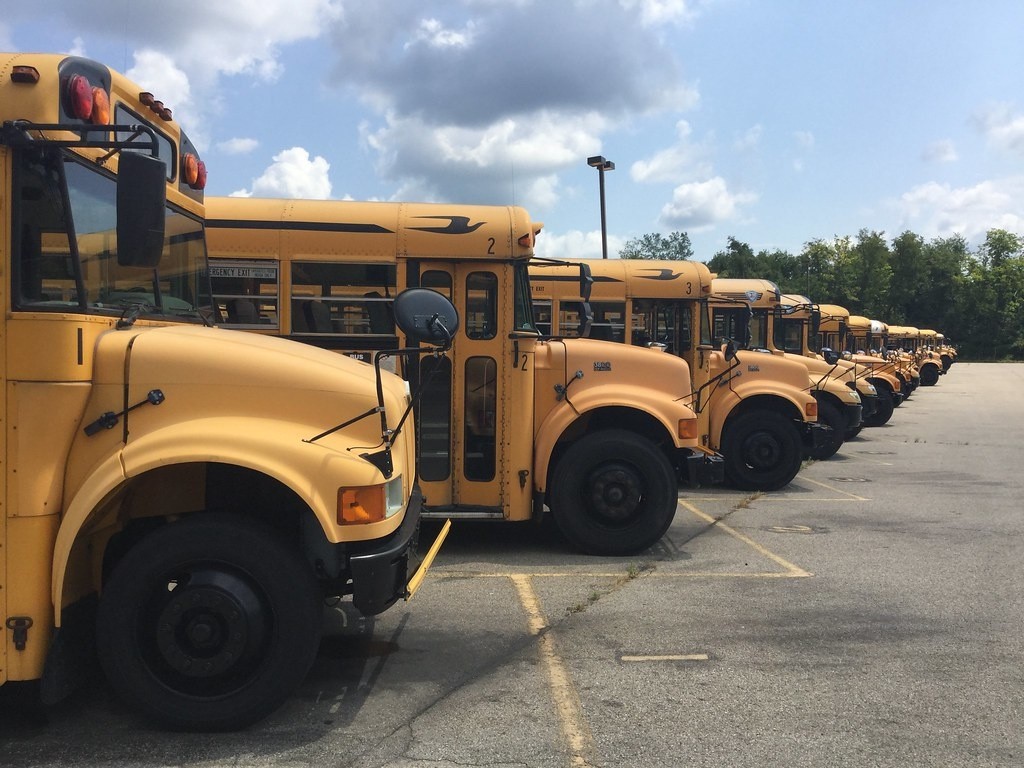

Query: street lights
[[585, 155, 617, 256]]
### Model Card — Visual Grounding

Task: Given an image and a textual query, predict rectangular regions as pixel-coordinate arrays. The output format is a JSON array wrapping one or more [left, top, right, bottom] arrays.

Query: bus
[[523, 253, 821, 493], [0, 50, 460, 732], [713, 272, 958, 461], [202, 193, 699, 556]]
